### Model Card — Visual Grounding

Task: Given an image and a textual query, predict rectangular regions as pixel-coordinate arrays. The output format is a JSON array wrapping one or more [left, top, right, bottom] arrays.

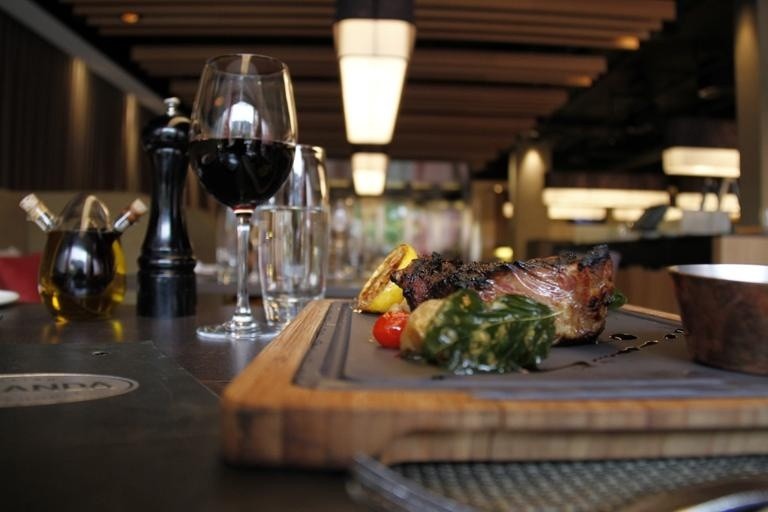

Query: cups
[[253, 144, 332, 329]]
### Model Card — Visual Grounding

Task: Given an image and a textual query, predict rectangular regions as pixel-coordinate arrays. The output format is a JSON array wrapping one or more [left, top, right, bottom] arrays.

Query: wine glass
[[189, 53, 298, 342]]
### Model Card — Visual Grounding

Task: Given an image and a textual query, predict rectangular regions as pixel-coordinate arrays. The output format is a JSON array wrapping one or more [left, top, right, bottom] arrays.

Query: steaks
[[402, 245, 616, 347]]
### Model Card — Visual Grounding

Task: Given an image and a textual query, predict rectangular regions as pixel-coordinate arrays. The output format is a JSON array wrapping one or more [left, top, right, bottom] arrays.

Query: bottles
[[18, 187, 149, 319]]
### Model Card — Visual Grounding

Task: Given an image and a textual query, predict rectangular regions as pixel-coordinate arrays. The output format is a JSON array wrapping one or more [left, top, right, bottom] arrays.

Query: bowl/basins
[[668, 264, 767, 375]]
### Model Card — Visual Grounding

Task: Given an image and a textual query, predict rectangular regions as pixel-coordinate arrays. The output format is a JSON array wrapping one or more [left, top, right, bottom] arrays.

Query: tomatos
[[374, 313, 409, 348]]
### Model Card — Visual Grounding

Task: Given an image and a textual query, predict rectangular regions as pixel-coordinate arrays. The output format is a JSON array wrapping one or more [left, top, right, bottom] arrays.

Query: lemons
[[358, 244, 418, 313]]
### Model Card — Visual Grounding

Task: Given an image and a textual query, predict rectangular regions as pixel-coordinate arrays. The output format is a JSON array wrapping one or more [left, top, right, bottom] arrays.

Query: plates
[[1, 290, 20, 305]]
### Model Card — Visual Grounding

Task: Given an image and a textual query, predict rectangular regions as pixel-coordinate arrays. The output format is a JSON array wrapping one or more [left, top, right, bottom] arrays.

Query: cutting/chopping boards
[[217, 300, 767, 466]]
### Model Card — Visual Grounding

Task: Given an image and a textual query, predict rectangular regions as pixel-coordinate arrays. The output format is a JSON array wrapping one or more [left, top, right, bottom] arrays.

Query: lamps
[[328, 8, 418, 145], [349, 151, 388, 198]]
